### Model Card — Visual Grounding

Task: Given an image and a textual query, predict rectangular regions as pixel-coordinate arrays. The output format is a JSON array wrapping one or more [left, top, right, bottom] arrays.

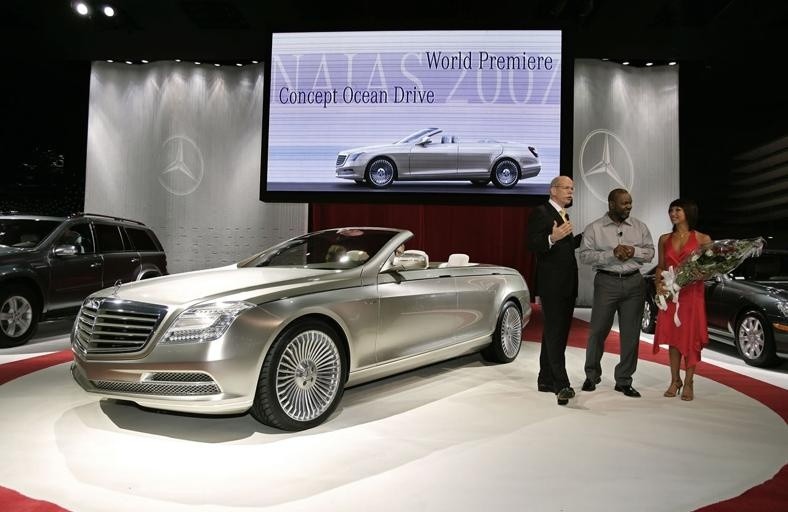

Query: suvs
[[1, 204, 177, 351]]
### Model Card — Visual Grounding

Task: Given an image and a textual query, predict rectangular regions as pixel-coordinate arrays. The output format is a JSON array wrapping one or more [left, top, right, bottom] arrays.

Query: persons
[[526, 175, 584, 404], [579, 188, 654, 397], [655, 199, 713, 402]]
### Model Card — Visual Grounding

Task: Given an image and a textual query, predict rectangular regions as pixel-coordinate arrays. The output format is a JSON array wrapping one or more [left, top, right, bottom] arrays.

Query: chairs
[[441, 136, 449, 143], [452, 135, 459, 143], [397, 253, 427, 270], [440, 254, 478, 268], [65, 232, 84, 253], [21, 234, 41, 242]]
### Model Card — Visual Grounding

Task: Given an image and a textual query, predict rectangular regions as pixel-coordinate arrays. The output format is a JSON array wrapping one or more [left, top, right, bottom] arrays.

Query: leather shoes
[[614, 381, 641, 398], [581, 376, 601, 392]]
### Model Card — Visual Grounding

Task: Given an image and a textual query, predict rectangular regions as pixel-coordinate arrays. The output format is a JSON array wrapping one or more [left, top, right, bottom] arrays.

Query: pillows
[[381, 252, 395, 271], [340, 251, 369, 262], [393, 250, 429, 267]]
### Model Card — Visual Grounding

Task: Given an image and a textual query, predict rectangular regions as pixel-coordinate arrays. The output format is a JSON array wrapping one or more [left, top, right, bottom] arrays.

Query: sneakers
[[538, 383, 555, 393], [557, 387, 575, 407]]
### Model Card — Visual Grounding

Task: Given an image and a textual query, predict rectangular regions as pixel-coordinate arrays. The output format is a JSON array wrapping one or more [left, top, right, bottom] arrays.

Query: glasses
[[553, 185, 576, 192]]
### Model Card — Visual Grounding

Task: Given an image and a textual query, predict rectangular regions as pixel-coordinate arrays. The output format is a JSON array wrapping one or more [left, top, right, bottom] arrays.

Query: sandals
[[680, 381, 694, 401]]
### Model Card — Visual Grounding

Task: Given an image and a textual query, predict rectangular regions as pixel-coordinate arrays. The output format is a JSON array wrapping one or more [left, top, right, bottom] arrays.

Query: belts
[[597, 268, 638, 278]]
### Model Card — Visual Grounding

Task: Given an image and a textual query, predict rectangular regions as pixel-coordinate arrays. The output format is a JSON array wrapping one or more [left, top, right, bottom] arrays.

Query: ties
[[560, 209, 567, 224]]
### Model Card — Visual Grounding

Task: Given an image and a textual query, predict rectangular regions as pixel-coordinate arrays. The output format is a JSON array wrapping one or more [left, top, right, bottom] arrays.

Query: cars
[[60, 220, 539, 437], [331, 123, 549, 195], [631, 228, 787, 374]]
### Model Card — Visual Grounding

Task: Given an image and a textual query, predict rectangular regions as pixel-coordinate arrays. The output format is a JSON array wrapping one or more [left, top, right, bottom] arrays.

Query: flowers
[[654, 237, 766, 303]]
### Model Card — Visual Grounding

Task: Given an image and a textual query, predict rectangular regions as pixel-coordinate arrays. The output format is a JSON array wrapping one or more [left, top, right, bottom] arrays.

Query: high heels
[[663, 380, 683, 398]]
